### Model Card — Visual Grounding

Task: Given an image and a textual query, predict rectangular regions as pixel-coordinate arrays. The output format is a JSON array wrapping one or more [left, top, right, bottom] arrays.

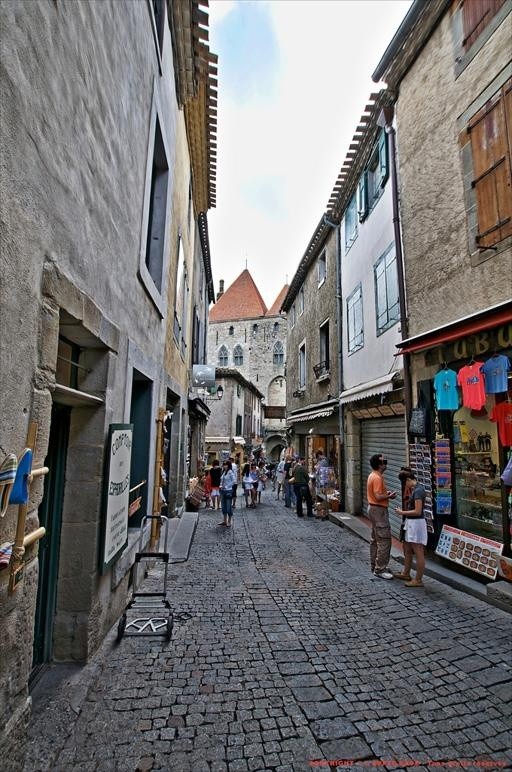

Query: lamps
[[205, 385, 223, 400]]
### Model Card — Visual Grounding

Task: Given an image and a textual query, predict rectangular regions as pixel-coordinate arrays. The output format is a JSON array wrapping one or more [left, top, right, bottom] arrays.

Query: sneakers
[[394, 572, 412, 581], [0, 452, 18, 518], [246, 503, 256, 509], [226, 522, 231, 527], [371, 567, 393, 580], [8, 448, 32, 505], [404, 581, 424, 586], [0, 542, 13, 570], [217, 521, 227, 525]]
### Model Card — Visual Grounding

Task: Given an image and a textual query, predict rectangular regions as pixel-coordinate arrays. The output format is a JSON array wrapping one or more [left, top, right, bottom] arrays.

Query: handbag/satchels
[[260, 473, 269, 482], [222, 489, 234, 499]]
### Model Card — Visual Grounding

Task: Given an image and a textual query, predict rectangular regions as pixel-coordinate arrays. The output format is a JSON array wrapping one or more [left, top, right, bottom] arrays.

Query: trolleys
[[118, 517, 174, 639]]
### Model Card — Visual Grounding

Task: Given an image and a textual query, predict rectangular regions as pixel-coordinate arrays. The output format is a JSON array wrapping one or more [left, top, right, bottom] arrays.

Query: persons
[[204, 451, 302, 510], [313, 450, 329, 470], [366, 453, 399, 580], [292, 456, 316, 517], [394, 467, 429, 587], [214, 460, 236, 527]]
